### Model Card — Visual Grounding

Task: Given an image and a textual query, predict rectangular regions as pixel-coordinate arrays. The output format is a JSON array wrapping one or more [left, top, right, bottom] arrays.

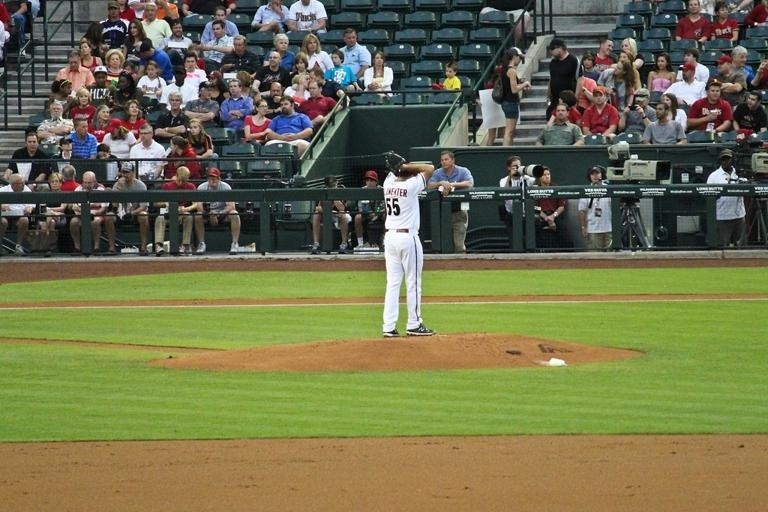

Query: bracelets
[[580, 225, 586, 230], [553, 210, 558, 217]]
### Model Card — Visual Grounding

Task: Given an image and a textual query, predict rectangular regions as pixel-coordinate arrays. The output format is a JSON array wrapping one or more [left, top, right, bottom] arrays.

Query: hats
[[588, 166, 602, 175], [509, 46, 524, 57], [706, 79, 722, 88], [593, 87, 606, 96], [199, 81, 214, 90], [94, 66, 107, 78], [175, 65, 186, 73], [677, 62, 696, 70], [108, 2, 119, 10], [547, 38, 565, 48], [105, 118, 121, 132], [633, 87, 650, 101], [207, 70, 222, 80], [716, 55, 732, 65], [718, 148, 733, 158]]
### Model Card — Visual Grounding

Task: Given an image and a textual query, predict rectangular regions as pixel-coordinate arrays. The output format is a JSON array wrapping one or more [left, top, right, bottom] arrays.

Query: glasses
[[635, 94, 647, 99]]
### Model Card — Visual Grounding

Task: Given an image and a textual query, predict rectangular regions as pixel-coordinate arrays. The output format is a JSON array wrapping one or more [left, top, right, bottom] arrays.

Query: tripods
[[614, 198, 652, 248], [738, 180, 768, 250]]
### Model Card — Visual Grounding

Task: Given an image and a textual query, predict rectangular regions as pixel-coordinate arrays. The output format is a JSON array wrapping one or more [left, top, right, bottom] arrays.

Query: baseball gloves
[[384, 153, 406, 177]]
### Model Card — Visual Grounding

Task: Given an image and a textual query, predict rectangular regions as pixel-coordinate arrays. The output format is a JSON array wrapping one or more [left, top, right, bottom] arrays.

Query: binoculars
[[517, 164, 544, 177]]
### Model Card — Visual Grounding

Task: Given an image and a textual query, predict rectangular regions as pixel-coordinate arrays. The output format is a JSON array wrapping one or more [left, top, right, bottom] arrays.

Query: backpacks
[[492, 67, 516, 104]]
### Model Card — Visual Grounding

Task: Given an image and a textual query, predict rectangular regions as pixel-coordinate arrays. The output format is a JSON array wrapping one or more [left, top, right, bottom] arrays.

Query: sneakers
[[154, 244, 165, 256], [383, 328, 400, 338], [179, 244, 192, 253], [311, 242, 321, 255], [0, 245, 149, 257], [405, 322, 437, 336], [339, 241, 350, 253], [195, 241, 207, 253], [229, 242, 239, 255]]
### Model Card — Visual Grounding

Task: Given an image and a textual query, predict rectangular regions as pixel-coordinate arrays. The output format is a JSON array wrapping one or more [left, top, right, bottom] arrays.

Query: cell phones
[[629, 106, 637, 110]]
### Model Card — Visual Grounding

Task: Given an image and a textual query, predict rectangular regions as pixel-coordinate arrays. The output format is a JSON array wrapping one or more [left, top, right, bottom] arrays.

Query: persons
[[426, 149, 473, 255], [704, 149, 748, 250], [1, 0, 393, 258], [432, 62, 461, 99], [531, 165, 567, 253], [378, 144, 435, 338], [578, 165, 615, 252], [499, 155, 544, 248], [476, 0, 767, 150]]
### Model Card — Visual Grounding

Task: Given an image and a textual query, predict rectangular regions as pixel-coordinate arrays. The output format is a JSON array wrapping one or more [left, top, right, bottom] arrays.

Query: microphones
[[599, 179, 603, 181]]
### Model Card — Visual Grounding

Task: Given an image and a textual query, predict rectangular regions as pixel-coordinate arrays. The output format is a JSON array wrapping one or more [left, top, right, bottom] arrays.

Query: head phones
[[587, 165, 607, 180]]
